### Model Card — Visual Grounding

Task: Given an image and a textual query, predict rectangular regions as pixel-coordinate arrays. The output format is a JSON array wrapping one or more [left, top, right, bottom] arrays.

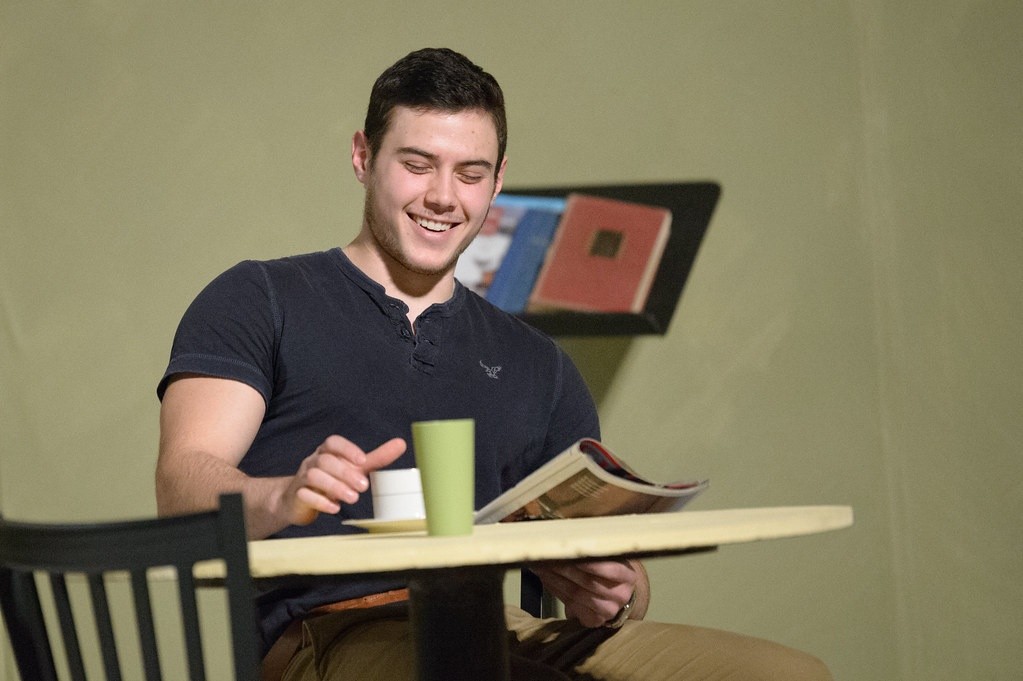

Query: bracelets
[[605, 591, 637, 629]]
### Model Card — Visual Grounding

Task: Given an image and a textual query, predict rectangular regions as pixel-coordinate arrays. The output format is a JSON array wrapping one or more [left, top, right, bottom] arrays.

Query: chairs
[[0, 489, 263, 681]]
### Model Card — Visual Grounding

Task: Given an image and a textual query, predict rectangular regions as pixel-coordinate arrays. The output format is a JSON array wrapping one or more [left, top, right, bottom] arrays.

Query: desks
[[40, 506, 854, 681]]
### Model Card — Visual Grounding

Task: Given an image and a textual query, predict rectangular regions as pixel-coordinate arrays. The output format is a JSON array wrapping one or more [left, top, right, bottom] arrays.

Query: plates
[[341, 517, 427, 534]]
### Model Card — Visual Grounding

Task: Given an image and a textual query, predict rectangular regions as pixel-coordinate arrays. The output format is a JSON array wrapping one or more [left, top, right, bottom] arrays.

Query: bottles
[[410, 418, 476, 536]]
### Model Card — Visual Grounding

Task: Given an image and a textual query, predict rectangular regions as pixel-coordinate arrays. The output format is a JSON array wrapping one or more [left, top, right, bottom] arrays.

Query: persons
[[454, 206, 513, 299], [156, 47, 833, 681]]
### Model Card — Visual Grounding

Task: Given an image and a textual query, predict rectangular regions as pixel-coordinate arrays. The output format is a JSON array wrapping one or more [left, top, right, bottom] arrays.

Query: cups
[[367, 467, 426, 518]]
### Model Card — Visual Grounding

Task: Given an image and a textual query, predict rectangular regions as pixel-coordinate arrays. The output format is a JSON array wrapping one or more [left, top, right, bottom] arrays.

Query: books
[[476, 439, 711, 524], [454, 194, 675, 314]]
